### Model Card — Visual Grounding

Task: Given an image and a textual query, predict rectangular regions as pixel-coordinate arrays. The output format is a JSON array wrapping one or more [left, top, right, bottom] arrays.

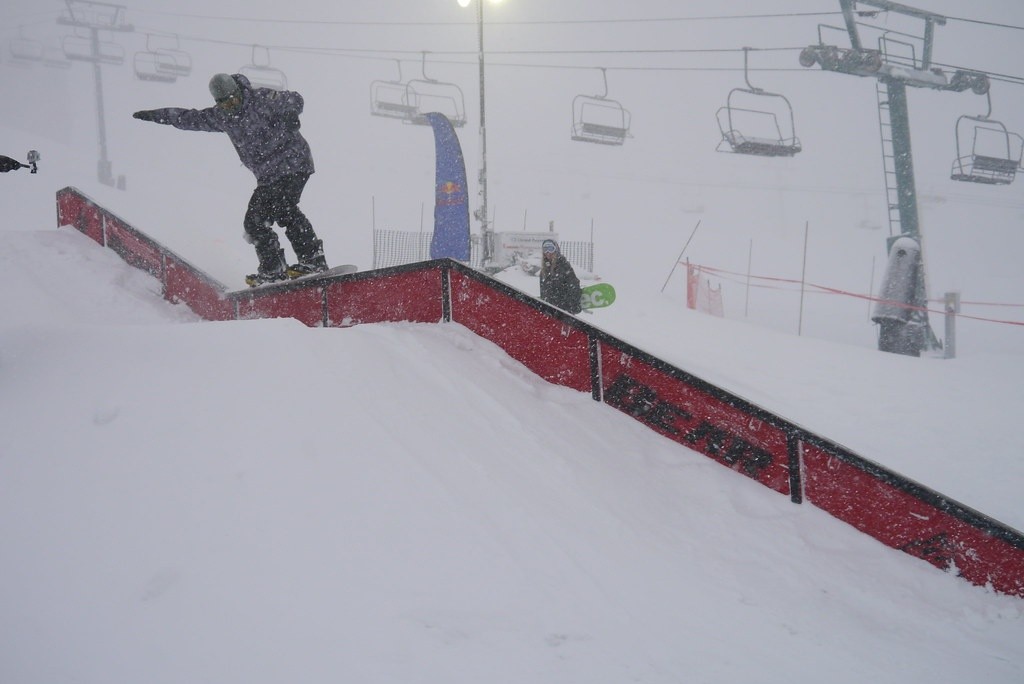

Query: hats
[[208, 73, 242, 109]]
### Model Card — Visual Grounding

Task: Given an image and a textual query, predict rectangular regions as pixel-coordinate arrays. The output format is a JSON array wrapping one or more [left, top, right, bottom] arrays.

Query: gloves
[[284, 111, 301, 131], [132, 108, 156, 126]]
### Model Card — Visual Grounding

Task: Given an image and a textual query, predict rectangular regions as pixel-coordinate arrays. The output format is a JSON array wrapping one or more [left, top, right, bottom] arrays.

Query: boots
[[245, 248, 290, 286], [285, 239, 329, 276]]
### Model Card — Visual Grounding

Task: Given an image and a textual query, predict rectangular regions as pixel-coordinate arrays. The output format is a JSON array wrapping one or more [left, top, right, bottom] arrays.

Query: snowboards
[[535, 282, 616, 314], [219, 263, 359, 303]]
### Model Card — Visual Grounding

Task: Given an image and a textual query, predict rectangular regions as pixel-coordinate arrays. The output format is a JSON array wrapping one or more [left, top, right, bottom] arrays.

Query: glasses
[[543, 246, 556, 253]]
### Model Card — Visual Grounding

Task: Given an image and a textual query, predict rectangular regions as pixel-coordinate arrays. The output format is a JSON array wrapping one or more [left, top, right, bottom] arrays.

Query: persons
[[132, 73, 329, 286], [539, 239, 582, 314]]
